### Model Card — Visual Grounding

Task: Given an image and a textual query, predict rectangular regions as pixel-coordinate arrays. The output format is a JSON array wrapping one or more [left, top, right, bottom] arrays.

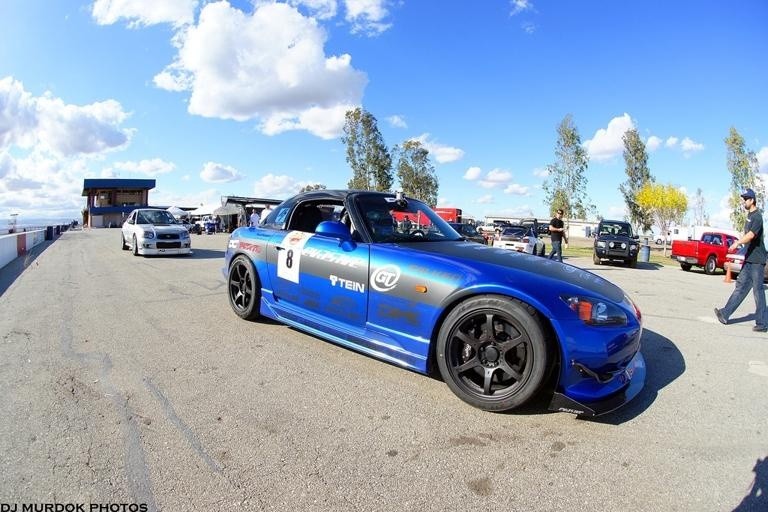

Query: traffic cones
[[722, 260, 734, 283]]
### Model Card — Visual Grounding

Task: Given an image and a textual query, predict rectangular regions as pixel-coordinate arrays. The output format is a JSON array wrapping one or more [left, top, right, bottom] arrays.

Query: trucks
[[391, 203, 462, 224], [651, 226, 729, 245]]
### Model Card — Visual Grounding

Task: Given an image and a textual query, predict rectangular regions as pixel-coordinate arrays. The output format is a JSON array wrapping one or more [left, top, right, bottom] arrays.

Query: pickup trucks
[[669, 231, 743, 276]]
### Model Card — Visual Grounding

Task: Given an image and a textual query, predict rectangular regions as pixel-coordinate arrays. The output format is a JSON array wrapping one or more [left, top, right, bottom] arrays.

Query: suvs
[[591, 216, 641, 267]]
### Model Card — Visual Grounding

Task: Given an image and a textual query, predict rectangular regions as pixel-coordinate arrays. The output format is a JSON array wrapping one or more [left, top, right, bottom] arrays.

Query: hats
[[740, 189, 755, 199], [557, 209, 563, 214]]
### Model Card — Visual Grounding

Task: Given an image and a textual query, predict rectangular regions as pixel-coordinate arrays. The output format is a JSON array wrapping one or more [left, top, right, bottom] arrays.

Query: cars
[[419, 214, 553, 256], [722, 246, 768, 282], [397, 219, 421, 228], [179, 215, 219, 233]]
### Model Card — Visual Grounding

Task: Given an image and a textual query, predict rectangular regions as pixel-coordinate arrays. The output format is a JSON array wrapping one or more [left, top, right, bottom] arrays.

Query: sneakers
[[714, 308, 728, 324], [753, 326, 768, 332]]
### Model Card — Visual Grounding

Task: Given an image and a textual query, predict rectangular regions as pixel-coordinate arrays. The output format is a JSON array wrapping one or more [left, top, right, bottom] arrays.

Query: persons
[[180, 211, 223, 235], [260, 203, 273, 223], [392, 216, 398, 230], [712, 188, 767, 331], [366, 210, 395, 242], [248, 208, 259, 227], [547, 208, 569, 263], [400, 214, 412, 235]]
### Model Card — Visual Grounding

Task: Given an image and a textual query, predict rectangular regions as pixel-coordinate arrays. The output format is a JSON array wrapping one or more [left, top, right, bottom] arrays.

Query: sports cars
[[222, 191, 647, 420], [119, 208, 193, 257]]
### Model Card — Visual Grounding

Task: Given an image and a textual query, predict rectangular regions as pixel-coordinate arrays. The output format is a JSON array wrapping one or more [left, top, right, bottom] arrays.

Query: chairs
[[293, 205, 322, 232], [601, 227, 609, 236]]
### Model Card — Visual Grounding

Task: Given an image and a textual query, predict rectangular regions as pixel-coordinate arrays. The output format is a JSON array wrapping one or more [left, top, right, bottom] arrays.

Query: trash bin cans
[[644, 238, 648, 245], [641, 245, 650, 262]]
